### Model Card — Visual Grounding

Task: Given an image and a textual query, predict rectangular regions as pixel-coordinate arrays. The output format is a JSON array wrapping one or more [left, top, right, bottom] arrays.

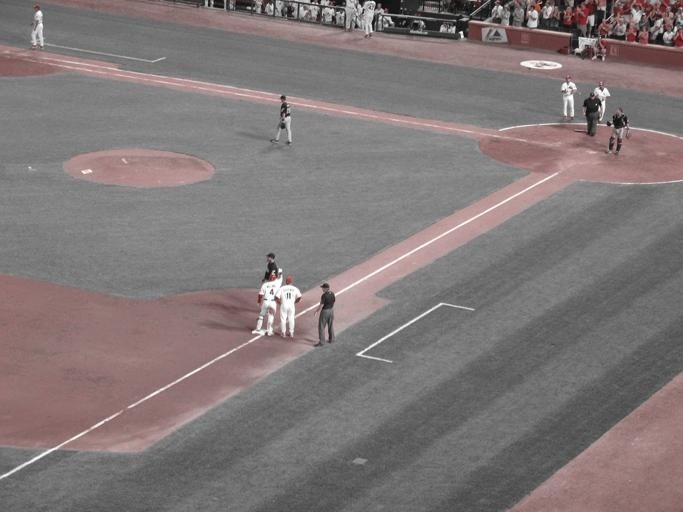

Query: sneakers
[[251, 329, 294, 338]]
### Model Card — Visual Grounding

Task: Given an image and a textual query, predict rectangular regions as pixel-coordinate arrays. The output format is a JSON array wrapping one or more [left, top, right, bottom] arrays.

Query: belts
[[264, 299, 275, 301]]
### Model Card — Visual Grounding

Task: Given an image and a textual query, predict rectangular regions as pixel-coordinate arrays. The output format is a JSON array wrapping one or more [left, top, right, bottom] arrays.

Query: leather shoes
[[314, 343, 323, 347]]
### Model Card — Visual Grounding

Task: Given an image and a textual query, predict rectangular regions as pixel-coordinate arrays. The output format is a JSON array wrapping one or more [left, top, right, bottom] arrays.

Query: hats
[[266, 253, 275, 259], [320, 283, 329, 288], [268, 275, 275, 281], [285, 276, 292, 284], [33, 4, 39, 10]]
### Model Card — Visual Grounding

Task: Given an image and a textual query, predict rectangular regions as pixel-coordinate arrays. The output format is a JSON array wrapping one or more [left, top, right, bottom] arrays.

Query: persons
[[593, 40, 609, 63], [261, 252, 279, 283], [606, 107, 630, 156], [269, 95, 292, 146], [492, 1, 682, 47], [28, 4, 45, 50], [312, 282, 336, 347], [440, 22, 456, 34], [247, 0, 427, 35], [582, 92, 603, 136], [593, 81, 611, 122], [560, 74, 578, 121], [251, 268, 284, 338], [274, 276, 302, 339]]
